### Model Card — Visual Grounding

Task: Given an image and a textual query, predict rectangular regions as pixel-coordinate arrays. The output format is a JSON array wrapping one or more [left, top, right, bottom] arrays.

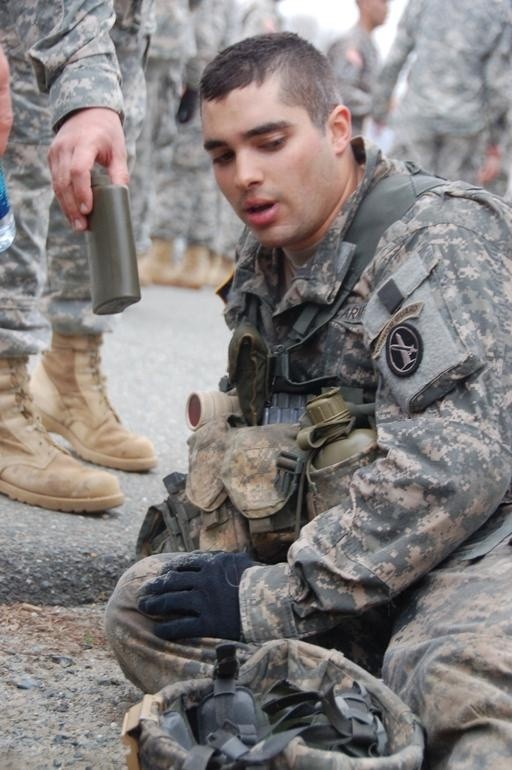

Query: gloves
[[177, 89, 197, 124], [135, 550, 264, 644]]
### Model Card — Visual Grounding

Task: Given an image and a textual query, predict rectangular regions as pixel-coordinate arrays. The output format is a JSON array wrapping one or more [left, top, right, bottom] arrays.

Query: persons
[[96, 21, 512, 770], [373, 0, 512, 192], [0, 1, 158, 516], [134, 0, 286, 294], [0, 48, 13, 158], [324, 0, 387, 139]]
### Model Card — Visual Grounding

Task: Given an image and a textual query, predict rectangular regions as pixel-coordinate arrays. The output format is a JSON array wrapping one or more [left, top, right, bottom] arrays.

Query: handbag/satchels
[[185, 421, 306, 561]]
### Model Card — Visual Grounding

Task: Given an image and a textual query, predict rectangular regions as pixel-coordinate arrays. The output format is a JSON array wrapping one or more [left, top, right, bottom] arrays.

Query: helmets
[[119, 638, 426, 769]]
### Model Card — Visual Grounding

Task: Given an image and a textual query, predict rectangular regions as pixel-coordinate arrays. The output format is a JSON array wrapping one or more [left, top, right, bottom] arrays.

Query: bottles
[[297, 397, 374, 469], [0, 164, 16, 252], [85, 167, 141, 315]]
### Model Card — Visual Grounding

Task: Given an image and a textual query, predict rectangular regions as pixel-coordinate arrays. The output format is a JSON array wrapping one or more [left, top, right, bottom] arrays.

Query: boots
[[0, 330, 158, 513], [137, 235, 235, 288]]
